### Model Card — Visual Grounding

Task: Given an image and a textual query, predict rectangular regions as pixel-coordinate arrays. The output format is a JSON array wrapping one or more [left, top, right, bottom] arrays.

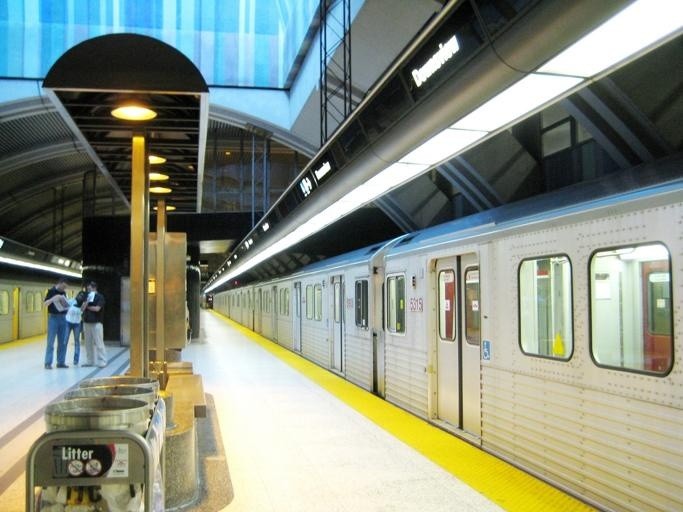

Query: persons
[[80, 280, 106, 367], [43, 278, 70, 369], [62, 290, 85, 364]]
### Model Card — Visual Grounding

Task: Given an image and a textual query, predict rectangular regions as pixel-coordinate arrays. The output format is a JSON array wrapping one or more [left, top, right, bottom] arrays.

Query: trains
[[210, 159, 680, 512], [0, 275, 87, 346]]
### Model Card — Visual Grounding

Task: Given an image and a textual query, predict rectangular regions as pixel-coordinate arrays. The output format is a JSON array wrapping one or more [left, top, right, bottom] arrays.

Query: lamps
[[109, 96, 176, 212]]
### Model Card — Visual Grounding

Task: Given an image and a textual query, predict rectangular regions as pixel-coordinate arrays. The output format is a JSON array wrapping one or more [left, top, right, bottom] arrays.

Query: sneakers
[[44, 360, 107, 370]]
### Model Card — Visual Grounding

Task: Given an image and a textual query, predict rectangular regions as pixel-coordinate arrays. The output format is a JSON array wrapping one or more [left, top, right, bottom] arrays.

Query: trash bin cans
[[43, 376, 161, 512]]
